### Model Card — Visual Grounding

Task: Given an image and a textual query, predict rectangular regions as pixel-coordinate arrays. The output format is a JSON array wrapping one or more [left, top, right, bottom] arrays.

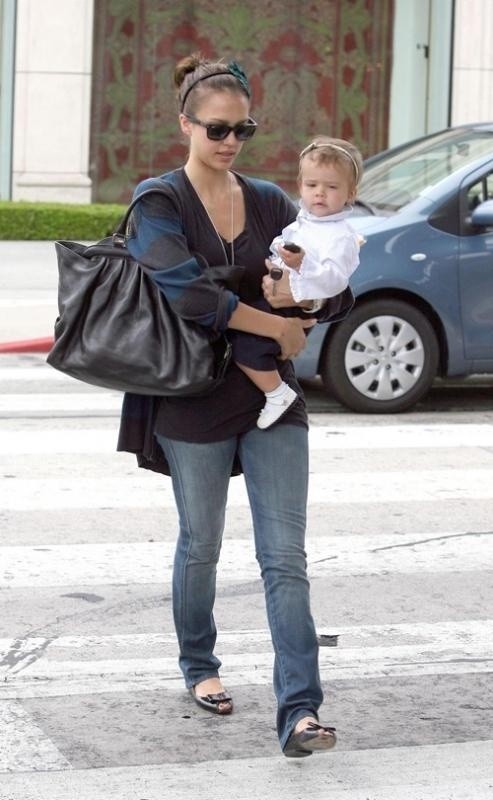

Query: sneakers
[[257, 383, 297, 429]]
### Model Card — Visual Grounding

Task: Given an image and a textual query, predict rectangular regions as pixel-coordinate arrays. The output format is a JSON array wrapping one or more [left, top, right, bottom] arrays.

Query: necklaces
[[184, 168, 234, 268]]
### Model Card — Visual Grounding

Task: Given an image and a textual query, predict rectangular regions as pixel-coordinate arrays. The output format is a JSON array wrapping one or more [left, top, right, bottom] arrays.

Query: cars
[[279, 120, 493, 415]]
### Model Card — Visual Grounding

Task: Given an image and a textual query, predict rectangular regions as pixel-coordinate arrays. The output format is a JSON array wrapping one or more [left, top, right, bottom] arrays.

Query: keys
[[269, 268, 282, 296]]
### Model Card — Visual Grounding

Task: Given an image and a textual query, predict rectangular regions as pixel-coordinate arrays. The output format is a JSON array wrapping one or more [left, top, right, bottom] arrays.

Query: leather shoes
[[189, 686, 233, 714], [283, 723, 336, 757]]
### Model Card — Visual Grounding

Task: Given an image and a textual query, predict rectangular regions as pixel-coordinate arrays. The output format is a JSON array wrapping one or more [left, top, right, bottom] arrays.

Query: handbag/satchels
[[47, 180, 245, 398]]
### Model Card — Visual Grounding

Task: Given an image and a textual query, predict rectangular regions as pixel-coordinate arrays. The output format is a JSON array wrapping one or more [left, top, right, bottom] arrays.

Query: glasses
[[188, 117, 258, 140]]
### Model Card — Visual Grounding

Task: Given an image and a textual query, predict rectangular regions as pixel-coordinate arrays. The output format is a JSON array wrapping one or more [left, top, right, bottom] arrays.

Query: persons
[[230, 135, 363, 430], [113, 54, 356, 757]]
